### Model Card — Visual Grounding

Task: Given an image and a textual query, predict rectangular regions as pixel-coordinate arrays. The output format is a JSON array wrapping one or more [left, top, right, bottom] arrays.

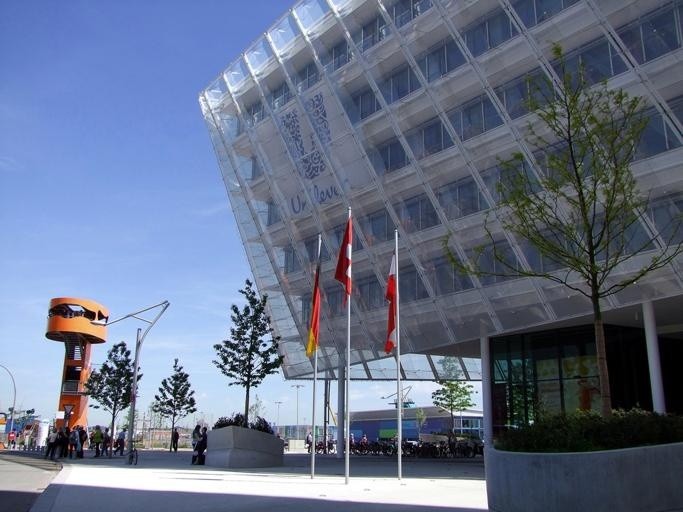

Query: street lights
[[290, 384, 305, 440], [273, 401, 283, 437], [89, 299, 171, 465]]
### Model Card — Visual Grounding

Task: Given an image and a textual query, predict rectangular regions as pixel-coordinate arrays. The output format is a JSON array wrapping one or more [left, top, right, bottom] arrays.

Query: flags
[[384, 247, 397, 353], [306, 239, 320, 358], [334, 217, 352, 305]]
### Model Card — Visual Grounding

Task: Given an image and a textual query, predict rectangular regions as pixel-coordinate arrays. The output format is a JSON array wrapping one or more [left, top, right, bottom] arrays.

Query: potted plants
[[439, 35, 682, 511], [202, 410, 285, 469]]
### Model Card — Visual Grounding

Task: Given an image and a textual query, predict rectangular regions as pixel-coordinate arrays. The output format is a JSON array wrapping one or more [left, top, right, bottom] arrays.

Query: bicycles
[[127, 439, 140, 465], [307, 438, 483, 459]]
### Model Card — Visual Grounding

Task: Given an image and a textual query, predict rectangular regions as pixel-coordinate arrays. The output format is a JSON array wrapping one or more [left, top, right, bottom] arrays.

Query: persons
[[197, 427, 207, 465], [192, 425, 203, 464], [8, 427, 126, 460], [173, 429, 179, 451], [307, 430, 483, 458]]
